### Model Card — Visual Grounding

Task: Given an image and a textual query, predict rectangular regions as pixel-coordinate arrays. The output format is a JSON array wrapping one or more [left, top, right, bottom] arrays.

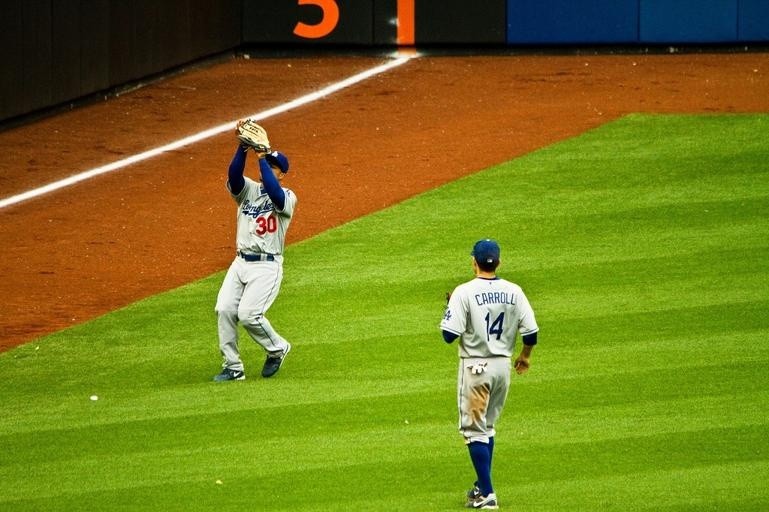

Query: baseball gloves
[[235, 119, 271, 155]]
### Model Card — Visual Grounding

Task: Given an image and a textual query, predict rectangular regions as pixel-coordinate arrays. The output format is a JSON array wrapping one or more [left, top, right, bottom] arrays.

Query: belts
[[237, 251, 274, 261]]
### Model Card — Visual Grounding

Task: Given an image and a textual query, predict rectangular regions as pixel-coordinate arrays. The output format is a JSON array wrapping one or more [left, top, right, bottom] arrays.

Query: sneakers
[[261, 343, 291, 377], [467, 487, 481, 501], [464, 492, 499, 509], [213, 368, 245, 381]]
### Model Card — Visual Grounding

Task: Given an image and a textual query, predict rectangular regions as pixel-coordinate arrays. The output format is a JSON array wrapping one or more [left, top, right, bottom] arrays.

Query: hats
[[265, 150, 288, 174], [470, 239, 500, 257]]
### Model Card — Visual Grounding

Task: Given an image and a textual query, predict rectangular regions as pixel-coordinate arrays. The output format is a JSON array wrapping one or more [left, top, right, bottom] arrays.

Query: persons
[[212, 116, 297, 382], [439, 238, 540, 510]]
